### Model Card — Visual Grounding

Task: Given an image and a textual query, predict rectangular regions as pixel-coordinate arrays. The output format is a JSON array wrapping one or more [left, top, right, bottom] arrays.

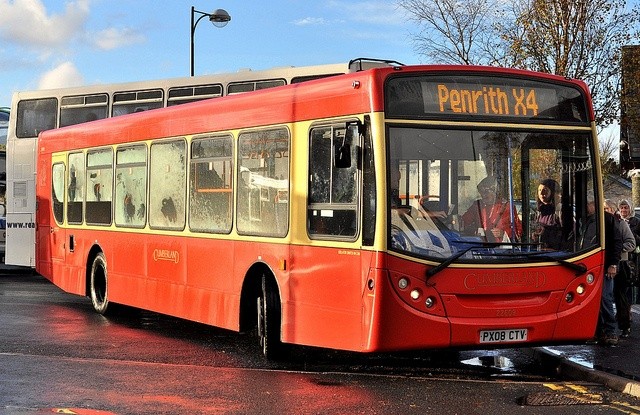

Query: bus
[[5, 56, 401, 270], [34, 63, 607, 363]]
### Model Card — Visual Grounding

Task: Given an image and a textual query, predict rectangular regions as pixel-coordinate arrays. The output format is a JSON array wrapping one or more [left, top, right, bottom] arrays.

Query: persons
[[531, 179, 577, 252], [363, 166, 448, 240], [618, 197, 640, 304], [461, 177, 522, 245], [604, 198, 637, 339], [582, 196, 624, 344]]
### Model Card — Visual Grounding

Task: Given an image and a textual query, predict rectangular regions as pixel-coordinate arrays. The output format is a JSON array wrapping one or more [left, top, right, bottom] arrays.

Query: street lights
[[190, 6, 231, 76]]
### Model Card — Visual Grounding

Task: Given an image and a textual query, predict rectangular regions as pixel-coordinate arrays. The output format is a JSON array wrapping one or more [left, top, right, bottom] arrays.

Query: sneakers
[[592, 335, 605, 340], [621, 328, 631, 337], [606, 335, 618, 344]]
